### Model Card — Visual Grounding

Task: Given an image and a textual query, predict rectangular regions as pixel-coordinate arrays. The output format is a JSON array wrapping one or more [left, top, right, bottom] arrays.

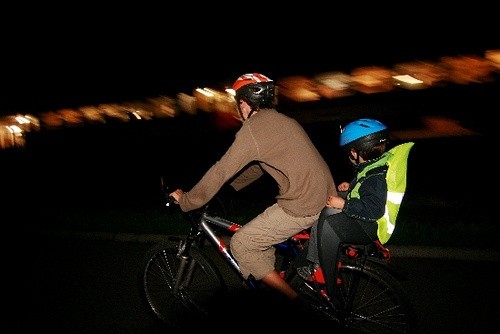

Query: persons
[[169, 72, 339, 304], [296, 118, 414, 286]]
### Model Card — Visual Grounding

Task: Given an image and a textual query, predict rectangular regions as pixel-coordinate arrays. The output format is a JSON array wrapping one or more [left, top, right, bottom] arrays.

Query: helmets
[[233, 72, 276, 107], [339, 118, 388, 151]]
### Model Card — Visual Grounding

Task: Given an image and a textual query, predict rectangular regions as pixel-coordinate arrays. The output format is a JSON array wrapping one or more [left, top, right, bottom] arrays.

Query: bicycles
[[142, 184, 408, 333]]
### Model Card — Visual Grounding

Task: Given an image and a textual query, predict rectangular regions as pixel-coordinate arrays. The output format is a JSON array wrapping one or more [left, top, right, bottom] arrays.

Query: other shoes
[[285, 294, 314, 317], [297, 266, 327, 286]]
